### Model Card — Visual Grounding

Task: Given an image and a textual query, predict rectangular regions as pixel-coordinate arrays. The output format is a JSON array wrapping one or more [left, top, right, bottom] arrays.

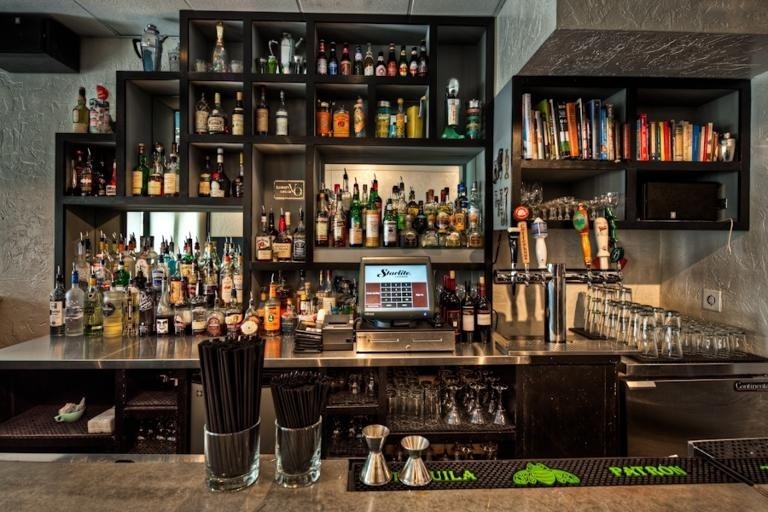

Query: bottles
[[401, 434, 431, 486], [257, 55, 278, 74], [315, 35, 491, 345], [358, 425, 395, 487], [50, 86, 315, 336], [211, 22, 229, 73], [169, 41, 180, 71]]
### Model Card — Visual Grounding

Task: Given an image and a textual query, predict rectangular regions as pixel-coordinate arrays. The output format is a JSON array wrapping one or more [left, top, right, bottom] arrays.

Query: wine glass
[[395, 440, 501, 460], [440, 365, 511, 429], [522, 177, 623, 219]]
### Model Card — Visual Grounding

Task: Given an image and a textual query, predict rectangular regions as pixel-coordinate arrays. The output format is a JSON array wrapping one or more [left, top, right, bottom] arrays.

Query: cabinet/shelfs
[[302, 362, 513, 460], [492, 75, 751, 232], [51, 10, 494, 331], [0, 365, 188, 454]]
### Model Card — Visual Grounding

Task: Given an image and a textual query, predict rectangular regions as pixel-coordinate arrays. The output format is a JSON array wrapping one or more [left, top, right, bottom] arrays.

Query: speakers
[[638, 181, 727, 221], [0, 12, 82, 74]]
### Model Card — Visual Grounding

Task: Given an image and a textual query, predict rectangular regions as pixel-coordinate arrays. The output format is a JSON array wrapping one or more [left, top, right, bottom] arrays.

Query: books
[[521, 87, 722, 164]]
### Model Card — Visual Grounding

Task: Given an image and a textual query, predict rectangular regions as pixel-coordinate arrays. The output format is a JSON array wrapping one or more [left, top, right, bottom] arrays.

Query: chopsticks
[[198, 335, 266, 434], [270, 370, 324, 426]]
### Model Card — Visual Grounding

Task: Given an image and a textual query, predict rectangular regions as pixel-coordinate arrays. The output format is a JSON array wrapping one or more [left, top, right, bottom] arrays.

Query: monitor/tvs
[[358, 256, 435, 329]]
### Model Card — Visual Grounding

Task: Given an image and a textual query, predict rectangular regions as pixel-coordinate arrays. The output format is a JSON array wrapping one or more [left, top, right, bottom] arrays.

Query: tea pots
[[268, 31, 304, 75], [131, 24, 168, 71]]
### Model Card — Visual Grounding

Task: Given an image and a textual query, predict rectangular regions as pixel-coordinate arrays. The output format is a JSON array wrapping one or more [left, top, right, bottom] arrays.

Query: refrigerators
[[192, 369, 281, 454]]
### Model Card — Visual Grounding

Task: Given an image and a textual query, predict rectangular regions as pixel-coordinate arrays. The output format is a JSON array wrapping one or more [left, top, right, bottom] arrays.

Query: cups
[[230, 60, 243, 73], [52, 408, 86, 424], [276, 416, 323, 487], [324, 369, 379, 456], [386, 376, 438, 428], [193, 58, 211, 73], [291, 55, 307, 74], [585, 282, 749, 360], [201, 416, 261, 491]]
